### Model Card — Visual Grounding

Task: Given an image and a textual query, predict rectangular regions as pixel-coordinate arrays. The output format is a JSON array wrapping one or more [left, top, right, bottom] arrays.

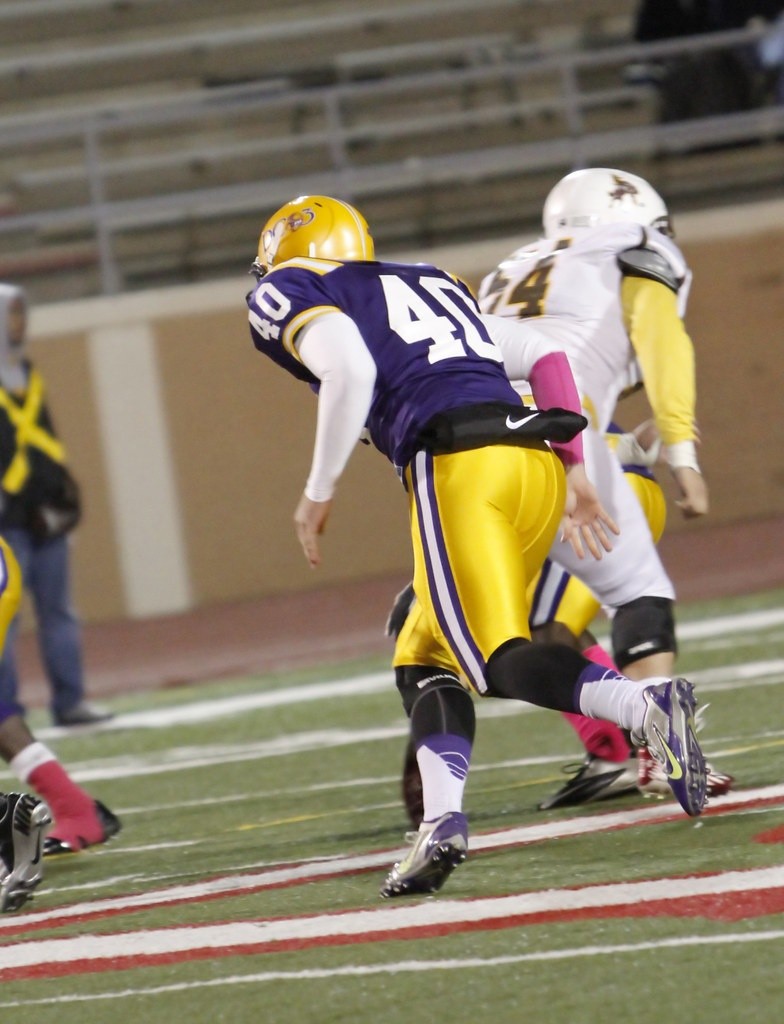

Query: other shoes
[[53, 701, 116, 726]]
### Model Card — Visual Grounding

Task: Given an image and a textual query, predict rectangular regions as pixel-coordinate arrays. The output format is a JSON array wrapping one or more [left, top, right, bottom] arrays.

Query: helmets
[[542, 168, 678, 243], [247, 196, 377, 279]]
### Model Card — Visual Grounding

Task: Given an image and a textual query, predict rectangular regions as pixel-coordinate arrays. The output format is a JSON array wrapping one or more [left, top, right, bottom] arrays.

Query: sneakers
[[0, 792, 56, 914], [635, 747, 671, 796], [538, 753, 639, 812], [700, 759, 735, 796], [630, 677, 709, 818], [377, 810, 469, 898], [43, 798, 122, 855]]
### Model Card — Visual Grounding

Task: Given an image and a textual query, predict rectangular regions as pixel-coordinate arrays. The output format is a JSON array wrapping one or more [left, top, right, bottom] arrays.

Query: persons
[[0, 537, 123, 916], [633, 0, 784, 152], [478, 167, 729, 810], [243, 196, 710, 898], [0, 286, 115, 727]]
